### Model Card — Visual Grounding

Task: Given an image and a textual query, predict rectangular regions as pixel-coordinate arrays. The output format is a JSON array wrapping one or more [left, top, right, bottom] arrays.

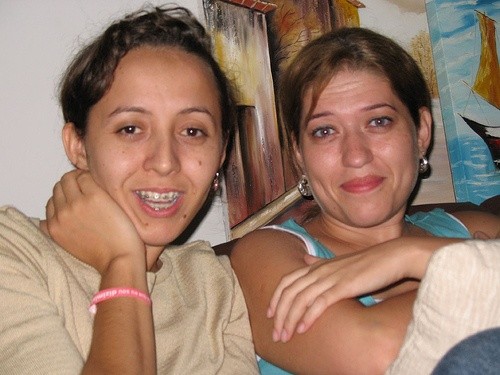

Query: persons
[[0, 1, 259, 375], [229, 27, 500, 375]]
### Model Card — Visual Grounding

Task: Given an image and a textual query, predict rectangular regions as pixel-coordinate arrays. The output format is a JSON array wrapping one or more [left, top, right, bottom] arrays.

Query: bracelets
[[88, 286, 154, 315]]
